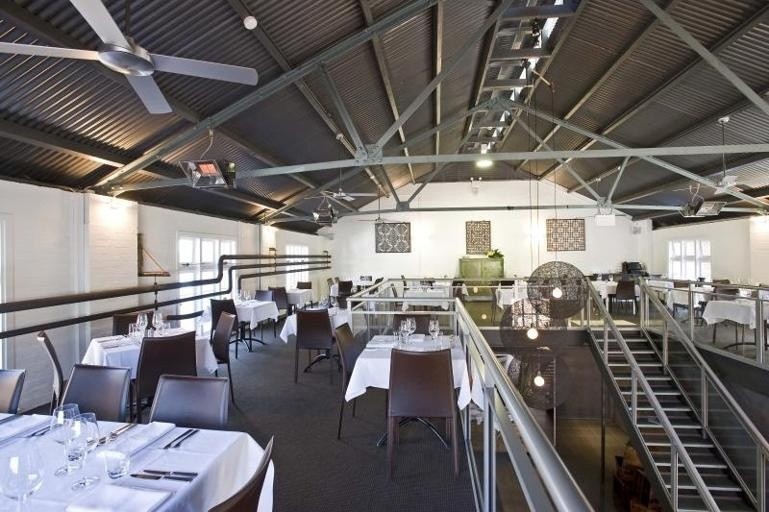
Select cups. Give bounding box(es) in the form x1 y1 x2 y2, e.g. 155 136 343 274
393 330 402 351
437 331 443 347
162 319 170 338
128 323 138 344
102 429 132 479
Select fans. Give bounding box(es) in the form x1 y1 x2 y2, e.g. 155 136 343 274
356 176 404 226
0 1 260 117
672 115 768 195
303 142 379 201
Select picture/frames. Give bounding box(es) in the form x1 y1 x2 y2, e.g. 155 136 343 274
546 218 586 251
465 221 491 255
374 222 411 252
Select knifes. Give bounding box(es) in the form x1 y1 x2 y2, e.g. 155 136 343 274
161 427 200 451
128 468 200 485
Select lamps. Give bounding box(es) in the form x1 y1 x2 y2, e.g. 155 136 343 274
230 1 259 32
526 83 588 318
311 197 339 225
177 128 237 191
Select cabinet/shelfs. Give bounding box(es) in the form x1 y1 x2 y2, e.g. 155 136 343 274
459 258 503 280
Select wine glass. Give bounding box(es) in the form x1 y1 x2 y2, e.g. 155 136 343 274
609 275 614 283
235 288 251 304
137 311 164 338
598 274 602 281
400 314 440 347
1 402 102 512
320 294 337 311
